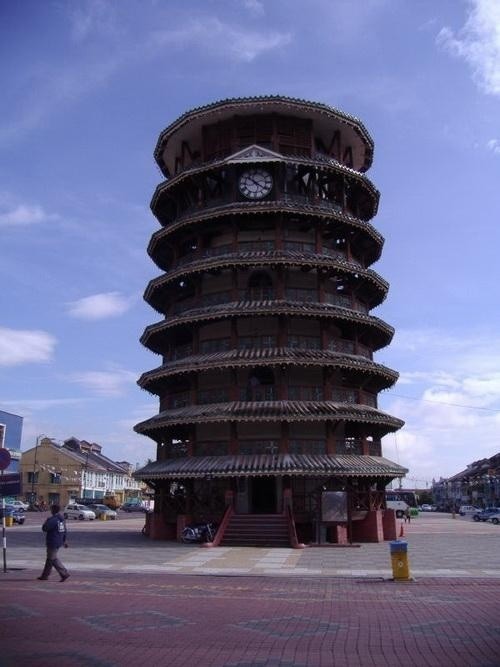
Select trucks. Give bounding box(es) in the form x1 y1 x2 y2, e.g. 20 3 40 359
75 495 121 510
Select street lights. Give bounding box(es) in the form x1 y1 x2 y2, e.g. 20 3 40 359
31 434 47 492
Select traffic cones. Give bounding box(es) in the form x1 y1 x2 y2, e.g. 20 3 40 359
398 522 406 537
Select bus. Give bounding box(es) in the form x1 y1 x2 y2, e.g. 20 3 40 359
385 489 420 517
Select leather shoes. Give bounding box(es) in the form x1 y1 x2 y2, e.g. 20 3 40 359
38 576 48 580
59 574 70 582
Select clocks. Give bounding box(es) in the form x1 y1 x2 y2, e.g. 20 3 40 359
238 168 273 200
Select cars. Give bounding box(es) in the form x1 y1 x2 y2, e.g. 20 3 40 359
123 503 149 513
63 504 117 520
0 501 29 524
420 504 500 524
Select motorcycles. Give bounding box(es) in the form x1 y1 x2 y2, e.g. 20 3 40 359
180 516 217 544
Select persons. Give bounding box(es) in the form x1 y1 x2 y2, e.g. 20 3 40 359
37 504 71 583
403 506 410 523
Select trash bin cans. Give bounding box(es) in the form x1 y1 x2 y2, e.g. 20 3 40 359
6 512 13 527
101 512 106 520
390 540 409 582
